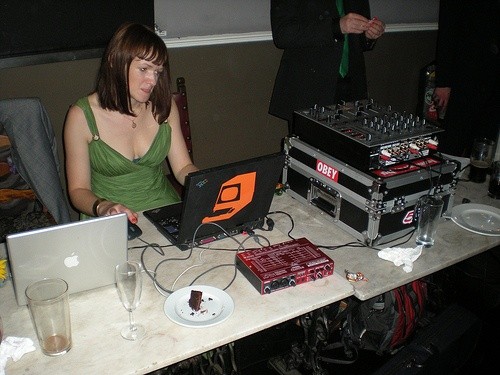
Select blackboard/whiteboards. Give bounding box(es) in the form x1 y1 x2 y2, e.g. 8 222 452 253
0 0 154 69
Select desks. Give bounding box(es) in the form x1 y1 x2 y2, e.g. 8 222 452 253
0 213 355 375
264 165 500 303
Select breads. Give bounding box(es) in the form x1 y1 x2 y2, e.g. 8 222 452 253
189 290 203 311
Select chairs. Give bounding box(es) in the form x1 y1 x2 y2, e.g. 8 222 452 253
0 96 73 242
165 77 195 167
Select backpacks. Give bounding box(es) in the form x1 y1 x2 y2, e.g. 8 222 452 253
346 275 426 356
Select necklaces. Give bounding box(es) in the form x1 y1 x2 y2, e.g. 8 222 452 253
116 102 148 128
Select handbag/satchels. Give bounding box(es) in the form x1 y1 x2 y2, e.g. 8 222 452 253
284 297 350 373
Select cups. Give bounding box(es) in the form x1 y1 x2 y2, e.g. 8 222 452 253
24 278 72 356
469 137 494 183
415 196 444 248
487 160 500 200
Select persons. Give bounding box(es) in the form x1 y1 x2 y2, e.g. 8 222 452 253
432 0 500 151
268 0 385 135
62 19 201 224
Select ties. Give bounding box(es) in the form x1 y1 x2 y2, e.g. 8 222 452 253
337 0 350 78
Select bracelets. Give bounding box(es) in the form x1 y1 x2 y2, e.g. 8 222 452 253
92 198 107 218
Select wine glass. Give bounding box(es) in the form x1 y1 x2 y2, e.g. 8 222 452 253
116 261 146 341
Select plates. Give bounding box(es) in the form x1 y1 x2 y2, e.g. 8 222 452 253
163 285 235 328
451 203 500 236
